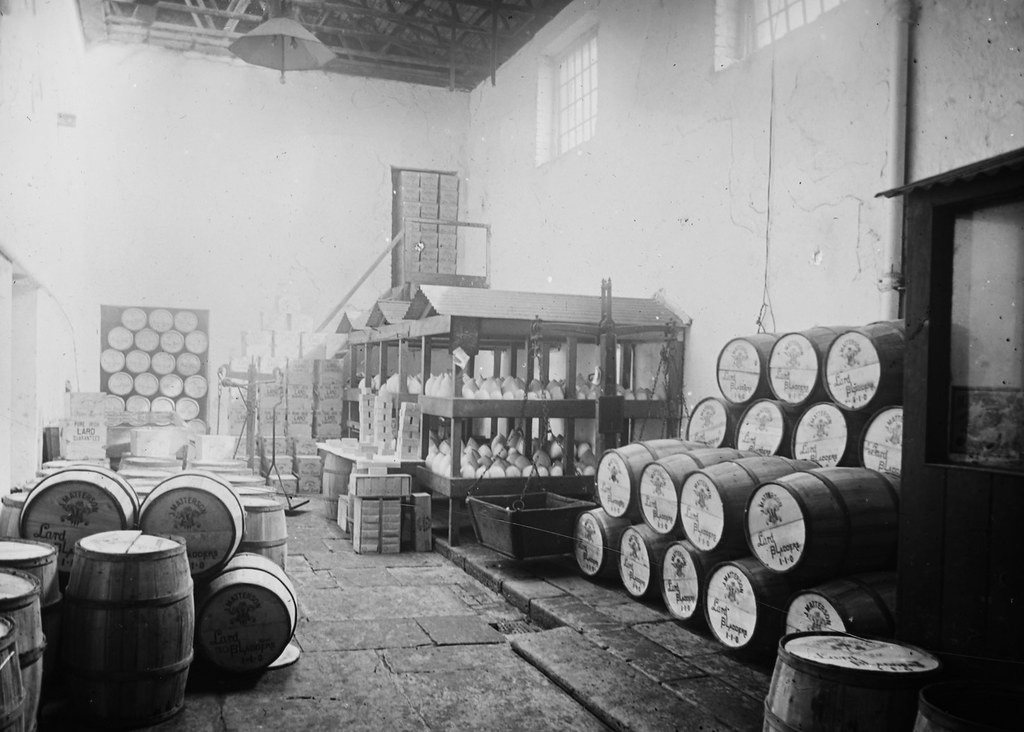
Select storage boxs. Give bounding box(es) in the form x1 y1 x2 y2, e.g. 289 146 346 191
247 359 432 554
58 392 107 460
195 435 237 461
129 427 179 460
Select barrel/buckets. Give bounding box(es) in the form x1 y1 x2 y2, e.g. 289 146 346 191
770 325 866 407
0 568 42 731
687 399 735 447
825 321 908 412
1 457 286 605
746 469 899 575
914 684 1024 732
639 449 765 535
663 542 724 623
716 332 778 404
762 632 940 732
574 509 630 580
792 404 861 469
861 407 903 487
787 587 938 639
1 614 23 732
598 439 706 518
197 552 296 672
620 525 679 599
682 456 819 551
738 398 792 457
705 558 804 650
67 530 195 716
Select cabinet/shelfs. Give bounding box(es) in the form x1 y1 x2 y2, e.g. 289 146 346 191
346 388 684 546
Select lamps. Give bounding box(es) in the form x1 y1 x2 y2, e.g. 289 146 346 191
226 0 338 85
